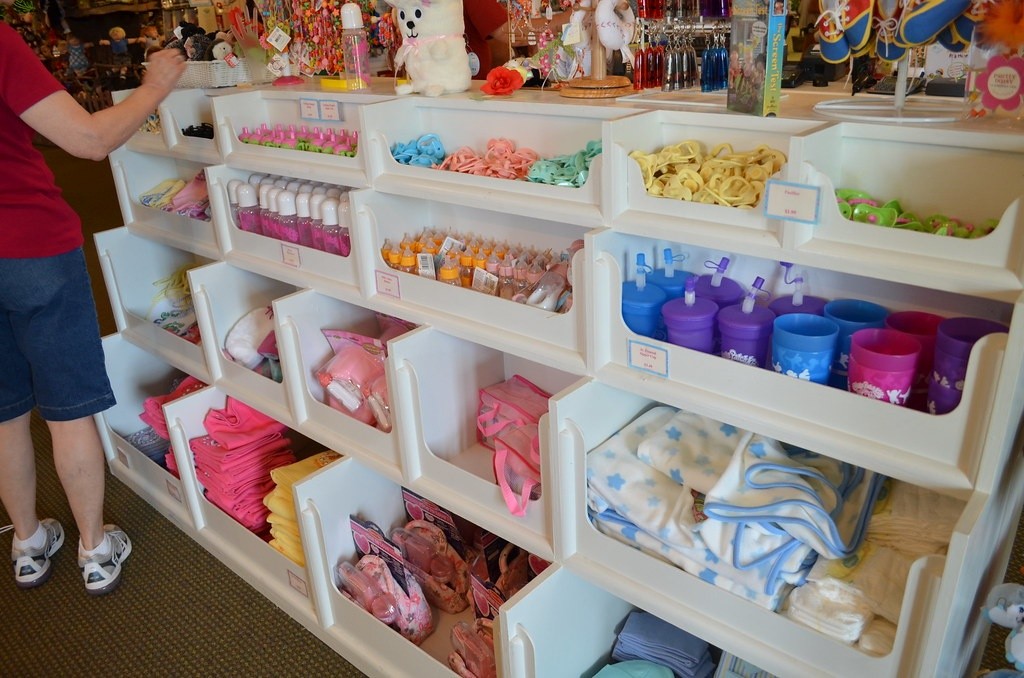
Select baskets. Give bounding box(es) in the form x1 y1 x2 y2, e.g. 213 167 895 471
142 54 291 88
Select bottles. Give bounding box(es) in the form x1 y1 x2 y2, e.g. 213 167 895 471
228 174 357 256
340 3 371 91
382 226 582 315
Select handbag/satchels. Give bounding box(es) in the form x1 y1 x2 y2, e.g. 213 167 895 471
476 374 555 517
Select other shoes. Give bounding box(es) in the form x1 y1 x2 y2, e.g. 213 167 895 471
813 0 991 88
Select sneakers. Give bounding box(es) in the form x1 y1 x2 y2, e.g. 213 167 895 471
0 518 64 587
77 523 133 595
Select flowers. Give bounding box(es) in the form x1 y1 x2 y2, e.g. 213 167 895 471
479 66 524 97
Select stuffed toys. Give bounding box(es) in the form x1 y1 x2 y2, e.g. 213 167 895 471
137 21 162 61
163 20 236 62
386 1 471 98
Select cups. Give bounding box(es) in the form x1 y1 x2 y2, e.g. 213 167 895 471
620 249 1007 415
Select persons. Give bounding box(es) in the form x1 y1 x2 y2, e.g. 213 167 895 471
97 26 140 81
62 33 94 74
0 20 188 595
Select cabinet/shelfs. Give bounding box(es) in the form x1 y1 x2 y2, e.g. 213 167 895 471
91 88 1024 677
